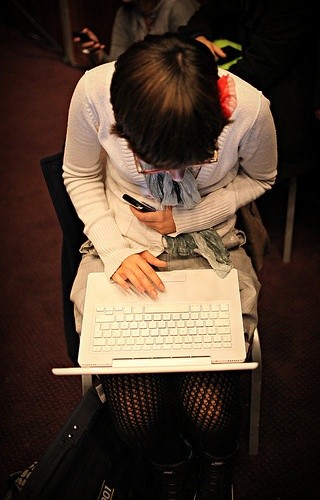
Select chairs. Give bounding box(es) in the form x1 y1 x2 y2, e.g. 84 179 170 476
40 149 262 455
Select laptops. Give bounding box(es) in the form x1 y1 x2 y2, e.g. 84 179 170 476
52 268 259 375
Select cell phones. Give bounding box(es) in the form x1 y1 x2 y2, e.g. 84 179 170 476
73 31 90 42
122 194 156 212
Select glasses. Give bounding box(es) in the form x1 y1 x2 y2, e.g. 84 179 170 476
132 141 219 175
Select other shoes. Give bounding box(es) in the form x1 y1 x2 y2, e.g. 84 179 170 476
195 439 237 500
151 448 192 500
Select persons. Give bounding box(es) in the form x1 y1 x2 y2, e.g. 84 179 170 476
178 0 320 161
72 0 202 73
62 37 277 500
